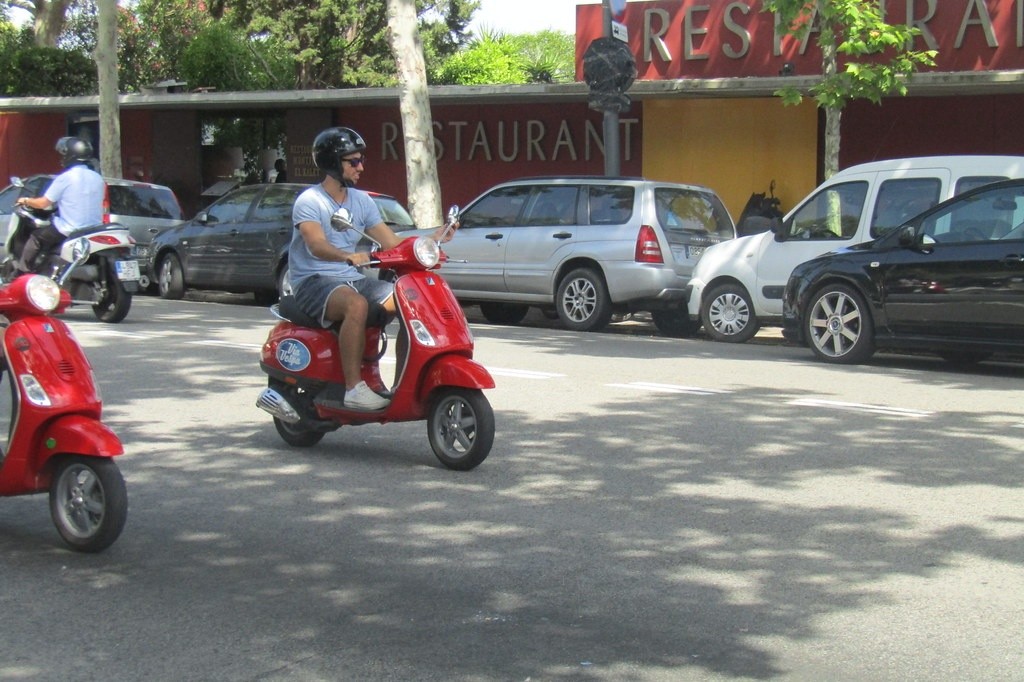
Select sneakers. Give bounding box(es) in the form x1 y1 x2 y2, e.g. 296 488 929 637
391 385 398 393
343 380 392 411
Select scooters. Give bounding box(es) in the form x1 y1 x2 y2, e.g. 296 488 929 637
737 180 786 239
3 175 150 323
256 204 496 471
1 238 128 553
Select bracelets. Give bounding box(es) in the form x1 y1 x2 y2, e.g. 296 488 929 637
24 197 29 205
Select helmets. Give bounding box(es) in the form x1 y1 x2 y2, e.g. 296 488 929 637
312 127 367 180
62 136 95 162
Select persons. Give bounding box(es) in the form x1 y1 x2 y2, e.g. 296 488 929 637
274 159 288 183
284 127 460 412
7 135 105 282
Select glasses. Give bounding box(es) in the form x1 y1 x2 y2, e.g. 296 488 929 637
340 154 365 168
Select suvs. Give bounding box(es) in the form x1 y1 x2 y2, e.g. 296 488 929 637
385 175 743 338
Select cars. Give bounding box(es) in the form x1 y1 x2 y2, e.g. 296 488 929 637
684 153 1024 368
146 182 418 308
0 173 188 296
